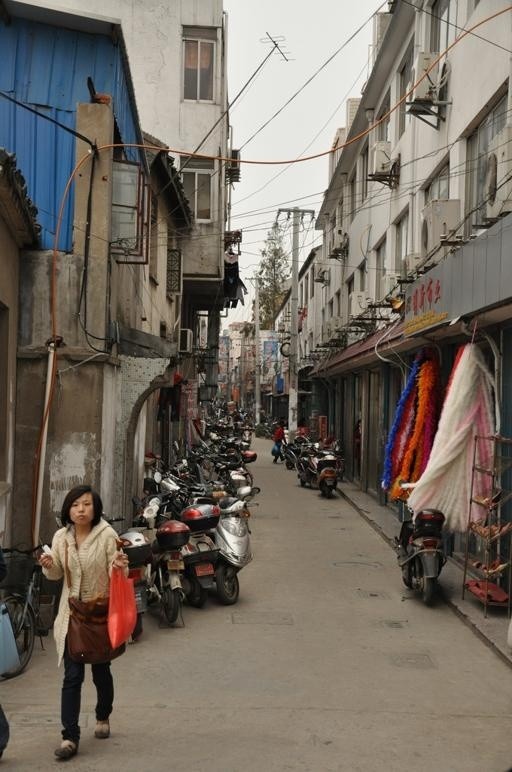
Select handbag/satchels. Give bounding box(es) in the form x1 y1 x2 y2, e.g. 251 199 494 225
67 594 128 663
0 605 23 678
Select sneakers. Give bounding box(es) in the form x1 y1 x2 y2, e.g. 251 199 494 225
50 738 79 760
94 719 111 741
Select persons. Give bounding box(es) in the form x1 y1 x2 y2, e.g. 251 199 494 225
0 544 11 761
232 411 240 435
273 422 288 464
38 484 130 758
240 411 247 422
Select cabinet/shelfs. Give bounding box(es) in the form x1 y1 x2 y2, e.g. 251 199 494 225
462 434 512 619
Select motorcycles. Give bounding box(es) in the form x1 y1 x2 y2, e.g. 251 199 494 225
282 434 345 498
261 413 285 439
393 497 448 606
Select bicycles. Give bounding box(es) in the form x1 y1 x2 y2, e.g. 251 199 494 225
1 544 52 679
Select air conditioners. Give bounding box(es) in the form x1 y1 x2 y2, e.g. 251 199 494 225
368 141 391 176
331 229 344 251
177 329 193 354
229 149 240 173
402 254 421 277
349 291 367 318
486 128 512 219
332 316 343 330
314 266 324 281
324 322 338 342
329 240 338 259
406 53 439 114
421 198 460 261
